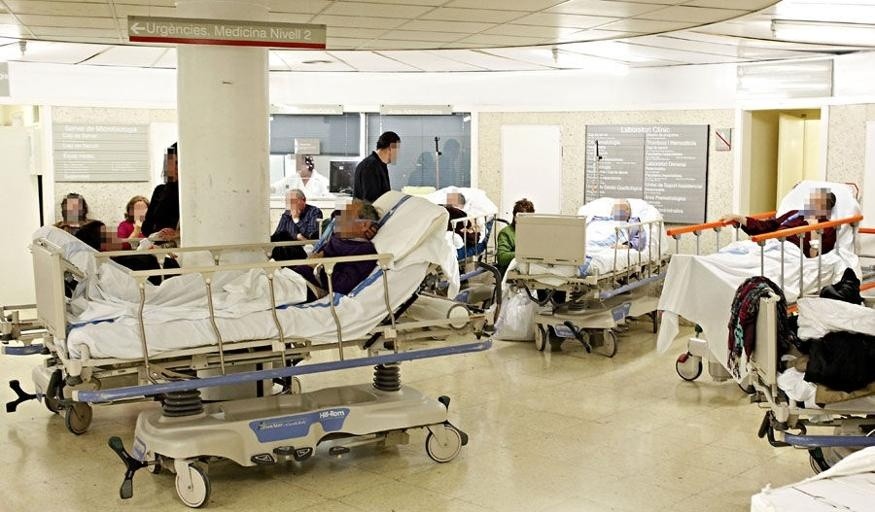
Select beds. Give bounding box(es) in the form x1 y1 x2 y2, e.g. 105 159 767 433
0 177 875 512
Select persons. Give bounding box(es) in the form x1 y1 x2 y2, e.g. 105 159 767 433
141 142 178 244
407 152 435 187
353 131 402 202
53 193 89 237
719 185 842 258
778 267 875 414
74 220 181 287
436 192 481 250
287 201 379 294
581 199 649 253
117 195 152 251
495 197 537 268
271 189 323 242
435 139 462 189
269 146 332 196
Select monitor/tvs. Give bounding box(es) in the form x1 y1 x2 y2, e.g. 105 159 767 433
329 161 358 196
